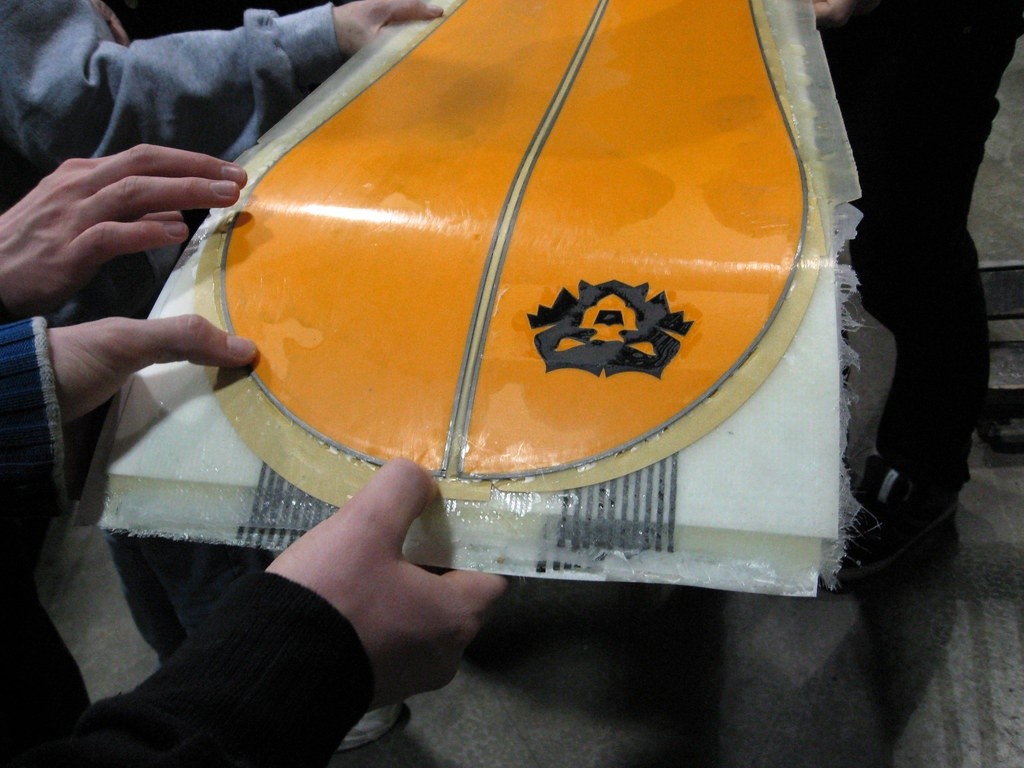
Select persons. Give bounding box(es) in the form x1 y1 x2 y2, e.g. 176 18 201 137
815 0 1024 593
1 2 506 768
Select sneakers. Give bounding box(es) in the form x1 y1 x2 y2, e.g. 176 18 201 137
835 455 959 579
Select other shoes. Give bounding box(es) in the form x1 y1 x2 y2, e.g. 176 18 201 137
336 702 410 753
